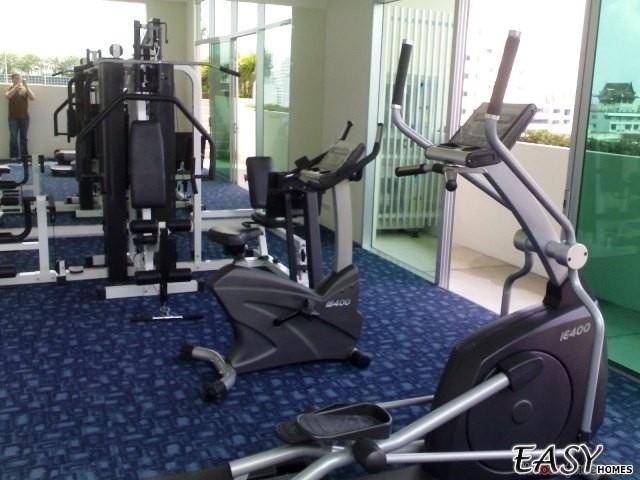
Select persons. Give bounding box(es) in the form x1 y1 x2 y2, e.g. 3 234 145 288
4 73 36 162
